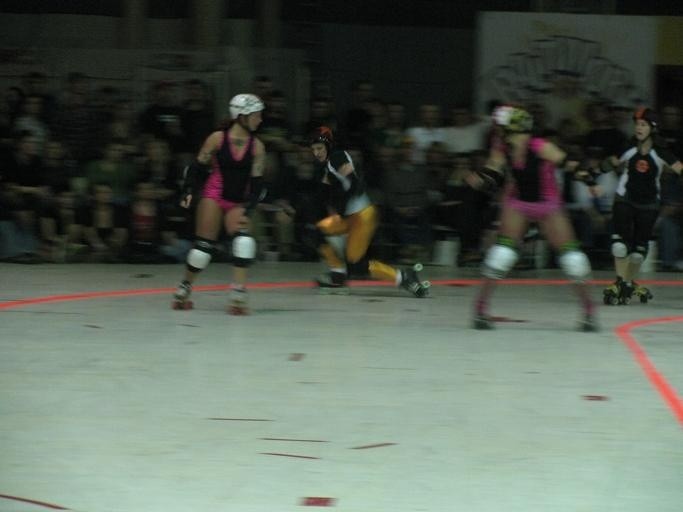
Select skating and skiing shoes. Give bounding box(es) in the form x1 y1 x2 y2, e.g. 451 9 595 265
168 279 195 312
399 262 431 299
629 281 653 304
225 286 249 315
312 272 352 296
470 312 599 331
601 283 634 306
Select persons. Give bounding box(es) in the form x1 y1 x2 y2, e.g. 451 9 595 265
305 124 428 299
424 105 607 329
171 92 267 311
583 106 682 300
0 71 683 275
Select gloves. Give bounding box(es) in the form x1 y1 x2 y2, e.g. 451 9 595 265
179 188 194 209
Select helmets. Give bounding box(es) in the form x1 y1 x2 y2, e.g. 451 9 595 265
305 131 333 146
226 93 265 120
632 106 660 128
493 106 534 132
